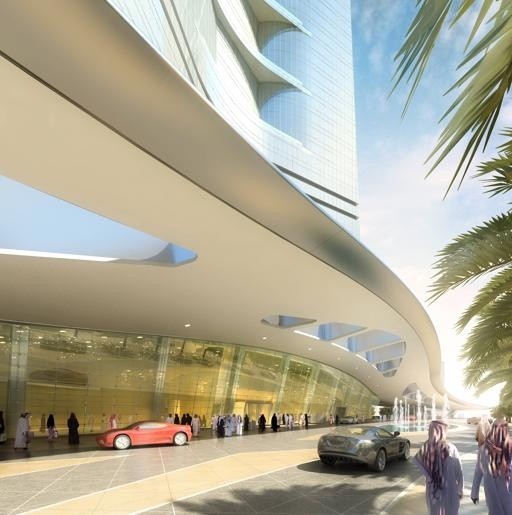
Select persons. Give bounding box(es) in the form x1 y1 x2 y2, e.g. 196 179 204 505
475 417 492 448
47 414 58 444
101 413 106 432
412 419 463 515
40 414 46 432
110 413 117 429
469 420 511 515
165 412 338 438
24 411 33 445
13 412 30 453
67 412 80 449
1 410 5 434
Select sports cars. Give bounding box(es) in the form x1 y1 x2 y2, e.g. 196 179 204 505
316 423 413 474
93 419 194 450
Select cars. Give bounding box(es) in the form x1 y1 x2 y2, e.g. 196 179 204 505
38 335 88 355
30 367 90 385
339 415 358 423
467 417 493 424
